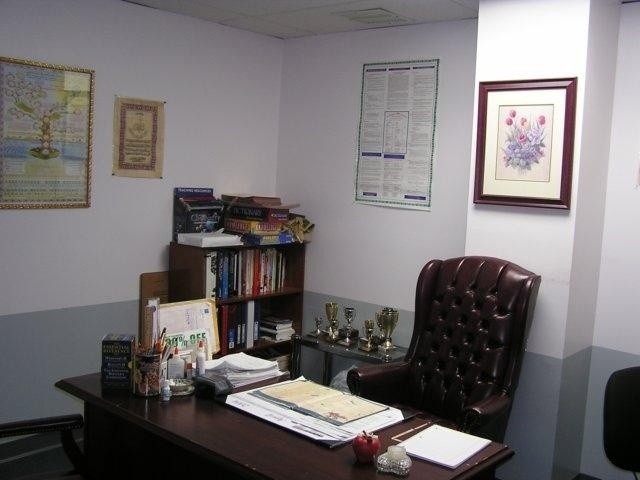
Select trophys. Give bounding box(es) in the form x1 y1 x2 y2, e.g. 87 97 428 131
309 301 399 354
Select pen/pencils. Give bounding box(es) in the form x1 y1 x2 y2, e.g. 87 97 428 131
152 328 169 354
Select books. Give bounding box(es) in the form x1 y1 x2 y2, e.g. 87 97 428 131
397 422 492 470
251 378 388 428
173 186 296 350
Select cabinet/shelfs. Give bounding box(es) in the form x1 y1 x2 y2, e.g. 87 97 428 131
169 241 310 370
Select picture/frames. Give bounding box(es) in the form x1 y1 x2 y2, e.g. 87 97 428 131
473 77 578 209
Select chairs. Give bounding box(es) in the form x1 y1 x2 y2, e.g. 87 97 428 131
604 366 640 480
347 256 542 444
0 414 85 480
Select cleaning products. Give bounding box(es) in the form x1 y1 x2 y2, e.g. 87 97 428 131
167 339 206 378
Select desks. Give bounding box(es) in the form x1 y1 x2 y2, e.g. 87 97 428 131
290 326 408 386
54 371 516 480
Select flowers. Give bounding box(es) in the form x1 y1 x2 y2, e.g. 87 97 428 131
502 109 546 170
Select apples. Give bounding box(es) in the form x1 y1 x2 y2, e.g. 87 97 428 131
352 430 380 464
